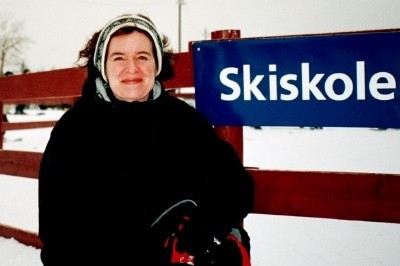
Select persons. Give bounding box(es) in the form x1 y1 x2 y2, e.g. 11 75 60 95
37 13 255 266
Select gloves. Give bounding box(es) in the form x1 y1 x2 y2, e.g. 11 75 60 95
177 202 236 256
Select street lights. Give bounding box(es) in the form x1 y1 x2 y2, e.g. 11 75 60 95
175 0 187 53
